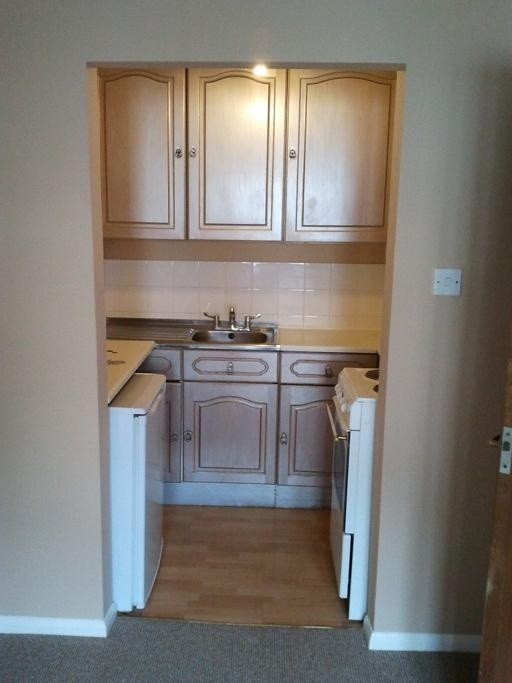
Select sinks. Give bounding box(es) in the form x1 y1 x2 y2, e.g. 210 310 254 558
185 328 278 346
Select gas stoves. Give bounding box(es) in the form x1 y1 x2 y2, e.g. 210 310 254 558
343 366 379 401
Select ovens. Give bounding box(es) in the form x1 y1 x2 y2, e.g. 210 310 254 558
324 395 350 533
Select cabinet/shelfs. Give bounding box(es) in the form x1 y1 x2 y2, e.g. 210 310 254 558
275 350 378 509
95 68 286 263
283 69 398 266
137 344 281 510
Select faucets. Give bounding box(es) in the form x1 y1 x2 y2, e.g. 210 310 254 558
228 306 235 324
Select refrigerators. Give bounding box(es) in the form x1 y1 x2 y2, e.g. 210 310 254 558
109 371 168 613
